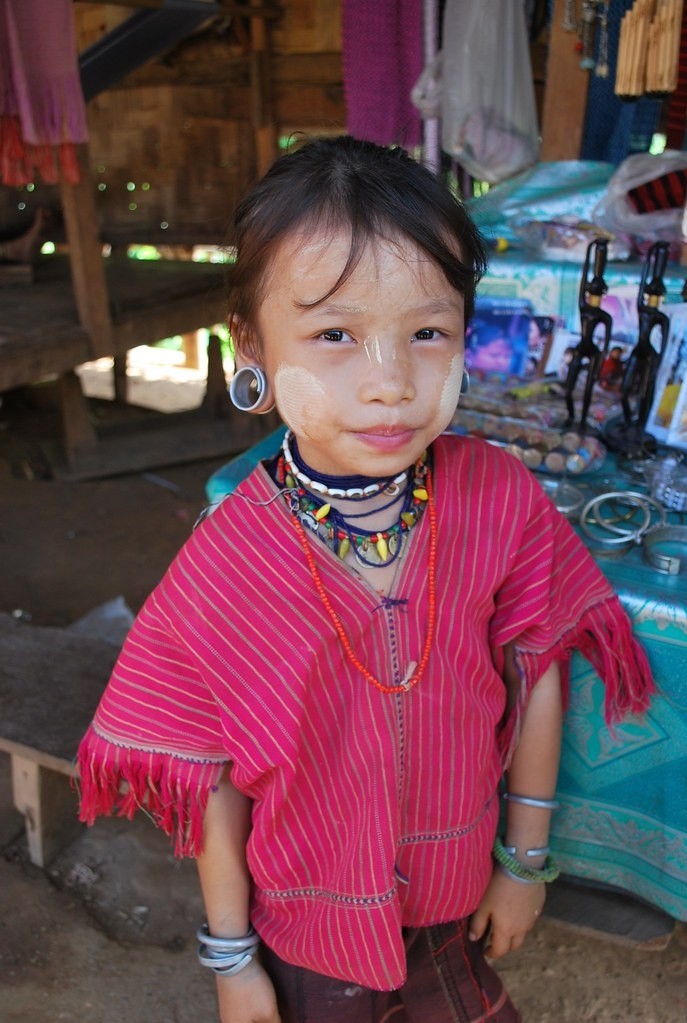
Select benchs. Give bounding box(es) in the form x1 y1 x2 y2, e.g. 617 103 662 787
0 625 124 867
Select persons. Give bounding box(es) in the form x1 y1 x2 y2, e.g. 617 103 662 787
562 237 612 433
599 346 625 391
619 239 671 436
556 345 575 382
72 135 656 1023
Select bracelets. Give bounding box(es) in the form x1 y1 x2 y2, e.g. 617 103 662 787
195 921 261 977
492 837 560 885
502 793 560 808
566 466 669 546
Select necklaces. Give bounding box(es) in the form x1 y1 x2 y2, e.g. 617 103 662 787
267 427 437 697
